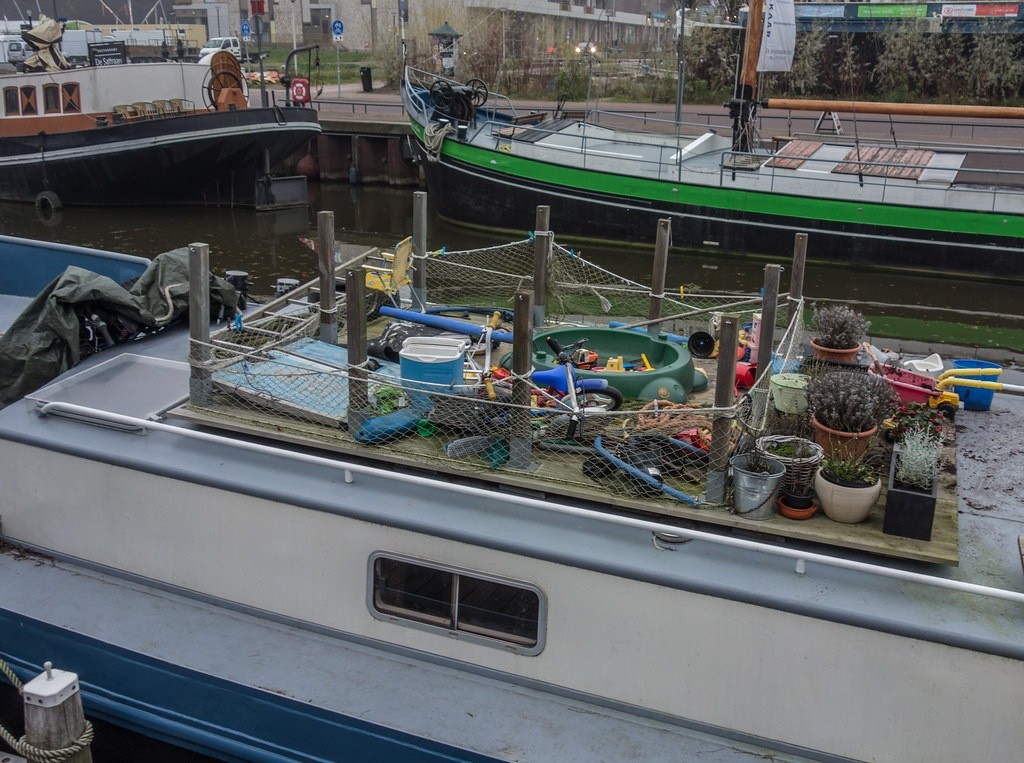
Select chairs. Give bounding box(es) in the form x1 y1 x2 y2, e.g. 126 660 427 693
113 99 196 123
361 235 427 316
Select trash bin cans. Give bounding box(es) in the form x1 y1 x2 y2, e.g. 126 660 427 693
359 66 372 93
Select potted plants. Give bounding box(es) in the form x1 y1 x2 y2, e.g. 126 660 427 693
729 441 786 521
810 303 872 363
803 367 901 460
880 424 948 542
782 418 816 509
814 419 884 524
755 435 824 489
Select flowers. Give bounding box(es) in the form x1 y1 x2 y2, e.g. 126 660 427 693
888 400 945 442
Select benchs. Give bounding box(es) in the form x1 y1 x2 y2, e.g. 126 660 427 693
491 112 548 149
556 111 590 123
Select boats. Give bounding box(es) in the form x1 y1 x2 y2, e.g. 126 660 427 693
1 0 322 207
403 0 1024 285
0 230 1023 762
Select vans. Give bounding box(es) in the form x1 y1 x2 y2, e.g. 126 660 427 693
7 40 36 61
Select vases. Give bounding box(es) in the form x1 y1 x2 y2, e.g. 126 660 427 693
776 496 817 520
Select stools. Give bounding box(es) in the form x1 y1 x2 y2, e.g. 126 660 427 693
771 136 799 154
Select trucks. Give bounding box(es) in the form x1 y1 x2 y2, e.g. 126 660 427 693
199 36 269 63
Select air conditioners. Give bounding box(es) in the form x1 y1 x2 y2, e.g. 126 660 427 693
915 17 942 33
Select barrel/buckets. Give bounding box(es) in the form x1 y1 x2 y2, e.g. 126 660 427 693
770 373 821 413
730 453 786 520
952 359 1003 410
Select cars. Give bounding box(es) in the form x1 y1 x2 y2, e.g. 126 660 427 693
576 41 596 54
